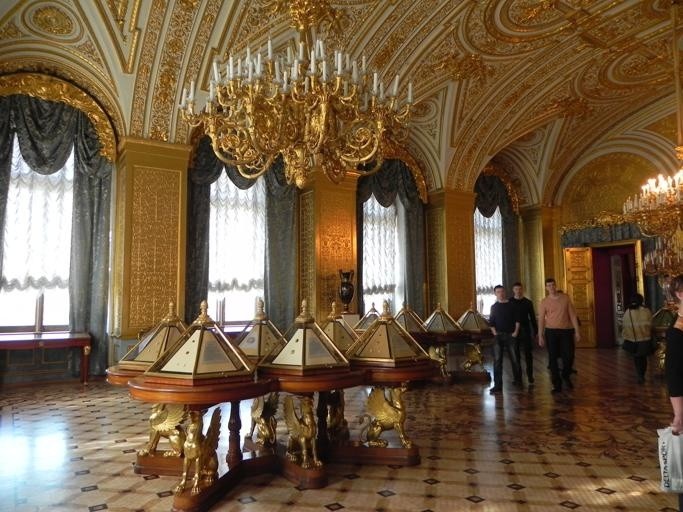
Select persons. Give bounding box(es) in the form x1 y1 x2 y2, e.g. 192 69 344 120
537 278 582 394
508 281 538 386
488 285 523 393
622 292 657 383
664 274 683 436
547 289 582 375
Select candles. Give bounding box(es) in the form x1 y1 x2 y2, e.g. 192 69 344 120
215 296 225 326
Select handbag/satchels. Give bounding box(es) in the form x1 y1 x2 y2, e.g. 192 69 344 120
623 339 637 352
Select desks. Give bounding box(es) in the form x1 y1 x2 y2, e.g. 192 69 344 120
0 331 92 387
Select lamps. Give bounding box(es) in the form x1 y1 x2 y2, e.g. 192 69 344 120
177 1 417 194
622 1 683 276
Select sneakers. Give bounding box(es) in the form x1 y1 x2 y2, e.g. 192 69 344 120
490 376 534 393
550 374 576 394
